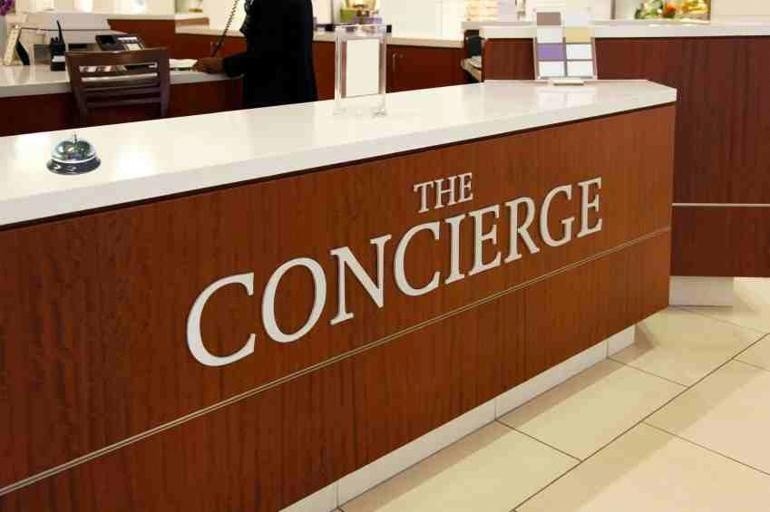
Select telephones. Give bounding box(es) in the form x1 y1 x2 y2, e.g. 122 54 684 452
95 35 155 71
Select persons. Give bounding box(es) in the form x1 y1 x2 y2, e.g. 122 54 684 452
194 1 317 111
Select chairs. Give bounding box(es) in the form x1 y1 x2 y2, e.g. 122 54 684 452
64 46 171 125
